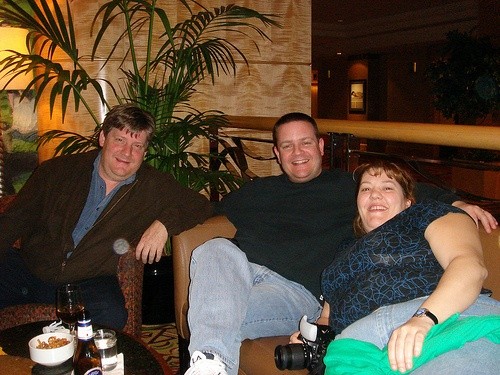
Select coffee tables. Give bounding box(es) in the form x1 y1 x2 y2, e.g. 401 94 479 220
0 321 173 375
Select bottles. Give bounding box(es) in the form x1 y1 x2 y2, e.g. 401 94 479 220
73 309 104 375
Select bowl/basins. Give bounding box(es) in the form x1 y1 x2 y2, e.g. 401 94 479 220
29 333 75 367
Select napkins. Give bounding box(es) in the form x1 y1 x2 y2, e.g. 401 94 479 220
72 350 124 375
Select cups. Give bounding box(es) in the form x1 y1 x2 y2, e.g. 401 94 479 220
93 328 118 371
360 143 368 151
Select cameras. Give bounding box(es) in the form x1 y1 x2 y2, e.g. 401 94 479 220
272 322 335 375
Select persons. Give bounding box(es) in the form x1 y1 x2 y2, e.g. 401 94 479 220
0 103 214 331
288 159 500 375
184 112 498 375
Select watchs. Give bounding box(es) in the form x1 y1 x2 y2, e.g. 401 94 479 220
412 308 438 325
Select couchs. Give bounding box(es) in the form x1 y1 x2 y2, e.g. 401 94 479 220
174 215 500 375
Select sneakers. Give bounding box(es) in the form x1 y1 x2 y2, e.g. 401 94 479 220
184 350 227 375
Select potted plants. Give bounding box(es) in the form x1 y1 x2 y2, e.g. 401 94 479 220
0 0 277 321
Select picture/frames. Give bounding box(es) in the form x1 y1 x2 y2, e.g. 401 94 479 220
349 80 366 113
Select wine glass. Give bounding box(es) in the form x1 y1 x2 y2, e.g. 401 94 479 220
57 284 85 333
